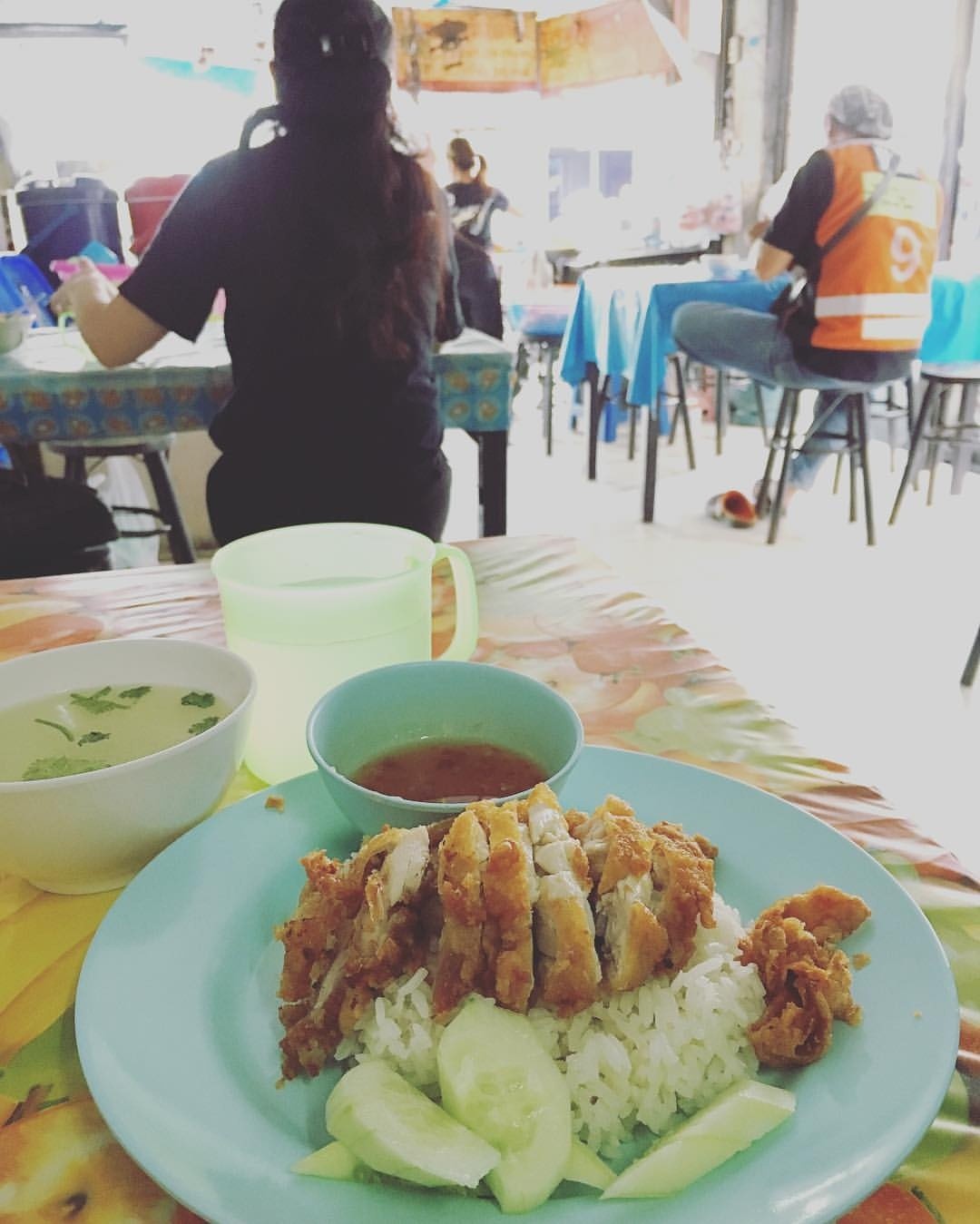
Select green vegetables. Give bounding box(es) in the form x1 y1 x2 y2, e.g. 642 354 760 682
7 676 222 792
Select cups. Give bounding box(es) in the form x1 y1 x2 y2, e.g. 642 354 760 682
211 521 480 793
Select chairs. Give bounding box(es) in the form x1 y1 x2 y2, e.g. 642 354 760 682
512 218 979 545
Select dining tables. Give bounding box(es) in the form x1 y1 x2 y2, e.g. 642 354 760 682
2 522 978 1221
0 292 514 536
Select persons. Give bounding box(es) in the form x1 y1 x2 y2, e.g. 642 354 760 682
437 136 521 343
669 80 945 516
55 0 464 553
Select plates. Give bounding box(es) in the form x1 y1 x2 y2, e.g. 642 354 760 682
76 743 961 1224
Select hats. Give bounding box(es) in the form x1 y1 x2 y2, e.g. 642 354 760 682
276 1 391 66
830 85 894 140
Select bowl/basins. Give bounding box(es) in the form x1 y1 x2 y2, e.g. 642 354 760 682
701 252 741 280
1 632 264 899
1 311 34 356
305 657 588 844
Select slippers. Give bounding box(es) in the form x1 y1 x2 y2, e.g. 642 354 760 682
706 489 756 528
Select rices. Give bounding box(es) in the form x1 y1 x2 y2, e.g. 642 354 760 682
309 884 774 1154
